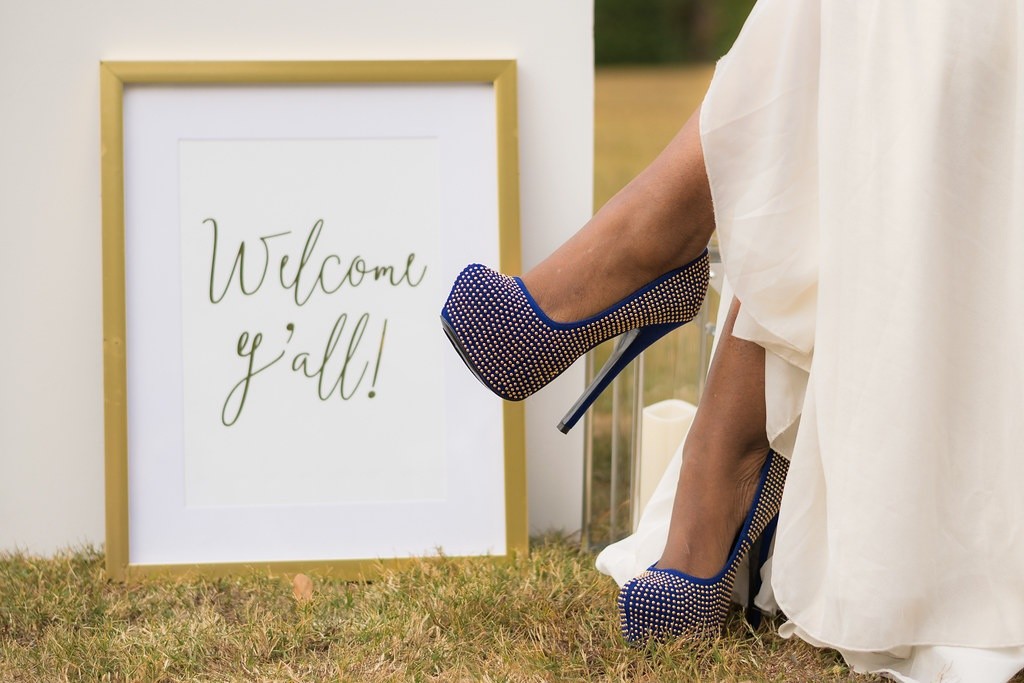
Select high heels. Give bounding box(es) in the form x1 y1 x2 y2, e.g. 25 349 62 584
619 449 791 646
437 246 711 434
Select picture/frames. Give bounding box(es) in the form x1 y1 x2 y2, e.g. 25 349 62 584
97 56 532 585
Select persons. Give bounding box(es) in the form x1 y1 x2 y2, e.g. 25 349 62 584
440 0 1024 683
